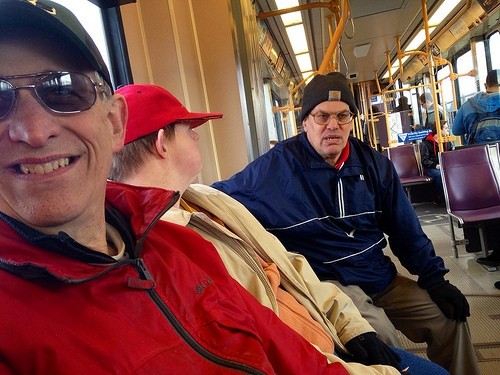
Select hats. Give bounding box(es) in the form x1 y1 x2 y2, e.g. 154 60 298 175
114 84 224 144
301 72 358 121
0 0 114 93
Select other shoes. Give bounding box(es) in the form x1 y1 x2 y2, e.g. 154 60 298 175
476 252 500 267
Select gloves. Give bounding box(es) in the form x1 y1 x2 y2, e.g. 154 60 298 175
345 332 402 372
425 274 470 322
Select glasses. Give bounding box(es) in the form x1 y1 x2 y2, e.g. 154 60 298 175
310 111 355 125
420 102 425 106
0 71 103 120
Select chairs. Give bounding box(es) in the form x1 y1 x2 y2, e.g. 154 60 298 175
377 112 435 209
438 140 500 271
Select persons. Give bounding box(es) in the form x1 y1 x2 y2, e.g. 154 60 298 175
420 120 456 207
391 96 417 144
451 68 500 174
363 104 382 146
209 71 480 375
474 221 500 293
411 93 446 143
0 0 352 375
109 84 448 375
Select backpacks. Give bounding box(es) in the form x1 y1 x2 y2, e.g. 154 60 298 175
464 96 500 145
